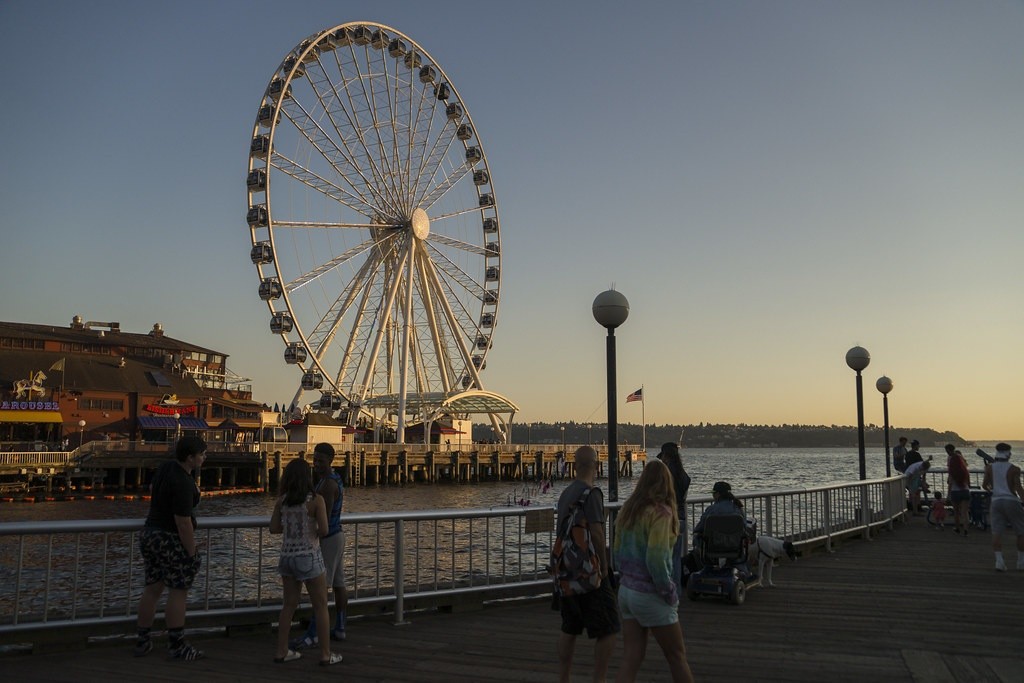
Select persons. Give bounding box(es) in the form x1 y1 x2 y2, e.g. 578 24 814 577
944 444 971 537
602 438 605 445
268 458 343 667
132 435 207 663
612 458 696 683
982 442 1024 572
555 446 622 683
289 443 346 653
893 438 908 473
58 437 69 452
419 437 427 455
903 460 930 513
103 431 111 441
472 437 503 453
693 482 753 578
8 442 15 452
445 439 452 452
655 442 691 597
905 439 923 467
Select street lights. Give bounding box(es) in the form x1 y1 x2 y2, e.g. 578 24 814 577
78 419 85 448
526 422 532 454
173 412 180 442
560 426 565 445
845 345 870 508
586 425 592 445
458 421 462 451
491 427 494 443
592 289 631 546
876 376 893 478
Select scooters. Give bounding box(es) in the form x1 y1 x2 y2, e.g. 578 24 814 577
686 517 761 606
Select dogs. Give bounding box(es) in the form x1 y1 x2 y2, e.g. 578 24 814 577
747 535 799 588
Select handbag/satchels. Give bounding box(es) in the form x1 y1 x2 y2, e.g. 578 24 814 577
546 485 606 597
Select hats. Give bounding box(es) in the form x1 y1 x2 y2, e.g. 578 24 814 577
656 442 677 458
995 447 1010 458
708 481 731 494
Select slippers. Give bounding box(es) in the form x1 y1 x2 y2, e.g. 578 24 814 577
273 650 302 662
319 652 343 666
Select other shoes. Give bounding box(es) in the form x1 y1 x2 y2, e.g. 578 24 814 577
1016 560 1024 569
996 561 1007 570
952 528 961 535
960 529 969 536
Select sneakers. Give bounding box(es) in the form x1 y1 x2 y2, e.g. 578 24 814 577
288 633 320 650
135 639 153 657
166 642 204 661
330 627 345 640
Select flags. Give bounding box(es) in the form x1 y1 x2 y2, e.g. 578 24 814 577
626 388 642 402
